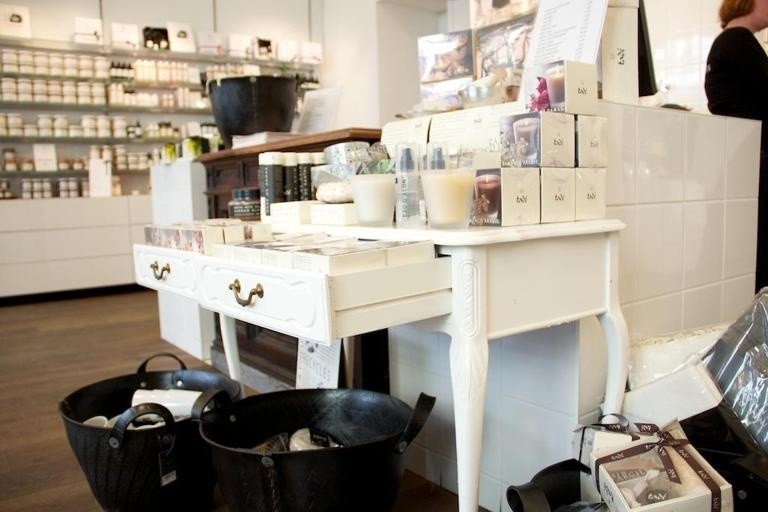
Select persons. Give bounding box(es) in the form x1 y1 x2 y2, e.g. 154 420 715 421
704 0 768 292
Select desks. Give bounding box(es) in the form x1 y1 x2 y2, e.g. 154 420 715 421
129 212 631 512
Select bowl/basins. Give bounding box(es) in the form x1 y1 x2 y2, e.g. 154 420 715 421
457 83 522 108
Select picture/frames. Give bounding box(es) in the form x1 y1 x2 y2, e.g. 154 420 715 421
472 9 538 80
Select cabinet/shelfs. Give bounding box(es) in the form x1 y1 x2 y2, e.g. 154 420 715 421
0 34 317 179
0 194 157 306
192 127 387 398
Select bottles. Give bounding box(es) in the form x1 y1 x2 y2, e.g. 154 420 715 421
228 188 263 223
110 58 203 171
395 144 427 229
112 176 124 199
204 62 255 95
259 150 325 216
0 49 113 202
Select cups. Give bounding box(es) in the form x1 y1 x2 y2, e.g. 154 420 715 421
544 66 567 113
348 174 397 227
475 174 500 222
512 119 539 165
